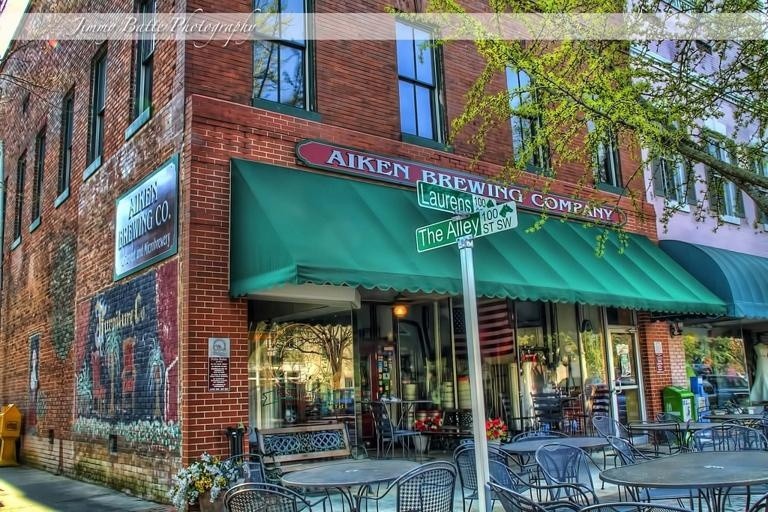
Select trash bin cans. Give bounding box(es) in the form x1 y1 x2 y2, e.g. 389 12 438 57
663 385 697 447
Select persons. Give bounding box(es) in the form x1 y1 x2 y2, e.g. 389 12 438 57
748 342 768 403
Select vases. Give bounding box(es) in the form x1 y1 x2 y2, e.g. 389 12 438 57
199 488 229 512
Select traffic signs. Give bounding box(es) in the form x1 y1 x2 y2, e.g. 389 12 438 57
414 202 519 252
417 181 499 219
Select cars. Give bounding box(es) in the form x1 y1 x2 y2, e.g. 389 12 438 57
263 383 403 423
547 371 751 419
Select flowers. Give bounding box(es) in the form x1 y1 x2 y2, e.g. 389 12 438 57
168 452 244 510
